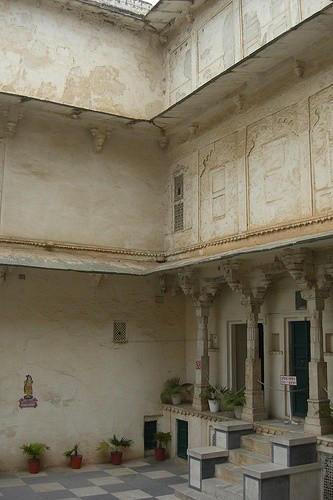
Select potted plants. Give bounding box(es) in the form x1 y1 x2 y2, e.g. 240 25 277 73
60 439 84 471
95 433 135 467
152 429 171 462
14 439 51 475
197 384 230 413
159 375 193 405
222 388 246 419
